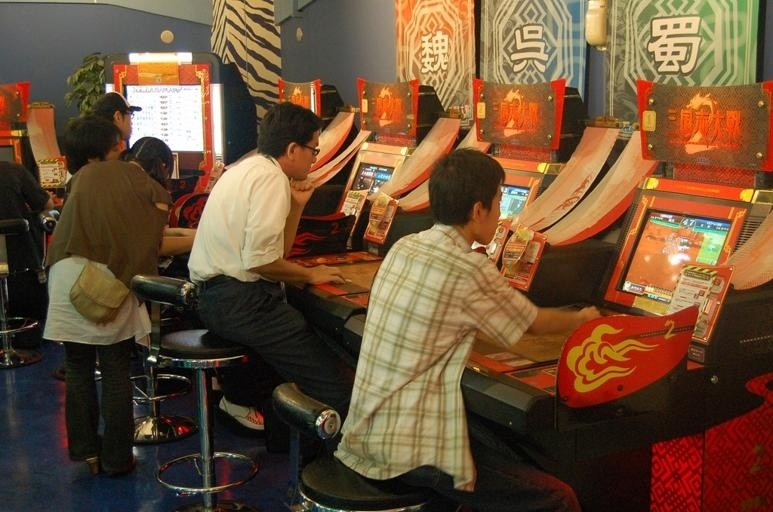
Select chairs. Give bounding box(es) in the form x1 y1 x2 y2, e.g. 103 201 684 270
0 219 43 369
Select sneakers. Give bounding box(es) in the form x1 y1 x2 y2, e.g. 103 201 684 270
211 376 265 430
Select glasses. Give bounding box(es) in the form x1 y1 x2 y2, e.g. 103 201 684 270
122 112 135 118
298 143 320 156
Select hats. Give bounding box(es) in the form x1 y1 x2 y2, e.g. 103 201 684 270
101 93 142 111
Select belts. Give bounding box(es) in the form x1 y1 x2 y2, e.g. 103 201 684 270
203 274 235 288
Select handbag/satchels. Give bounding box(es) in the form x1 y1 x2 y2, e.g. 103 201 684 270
69 264 130 323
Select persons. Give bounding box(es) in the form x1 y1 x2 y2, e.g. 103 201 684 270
0 161 54 350
64 118 264 430
332 148 602 512
187 102 353 456
91 93 142 140
42 137 174 477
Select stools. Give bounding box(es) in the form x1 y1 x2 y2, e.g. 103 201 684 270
129 272 259 511
272 382 427 512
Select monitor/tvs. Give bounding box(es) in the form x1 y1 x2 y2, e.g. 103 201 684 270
621 211 731 303
350 163 395 195
0 146 14 163
499 184 530 222
